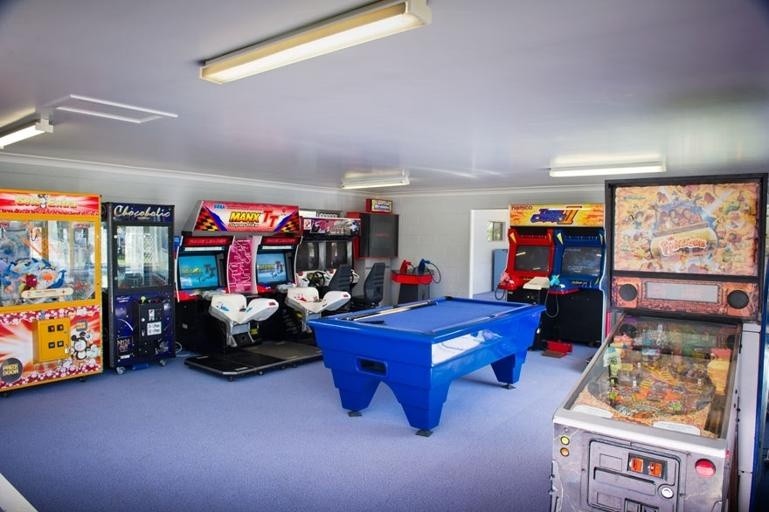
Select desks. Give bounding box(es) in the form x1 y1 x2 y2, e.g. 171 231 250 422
306 295 547 437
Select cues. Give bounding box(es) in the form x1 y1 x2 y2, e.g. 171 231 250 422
344 300 438 321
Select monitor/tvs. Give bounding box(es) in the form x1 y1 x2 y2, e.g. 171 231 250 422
326 241 347 269
361 213 398 257
562 246 600 276
255 252 288 281
177 254 221 290
514 244 549 271
296 241 320 271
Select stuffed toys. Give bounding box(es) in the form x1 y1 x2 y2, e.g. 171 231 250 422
0 256 67 305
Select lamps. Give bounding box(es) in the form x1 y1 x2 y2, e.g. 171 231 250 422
199 0 433 86
338 172 411 191
0 119 54 150
548 160 667 178
